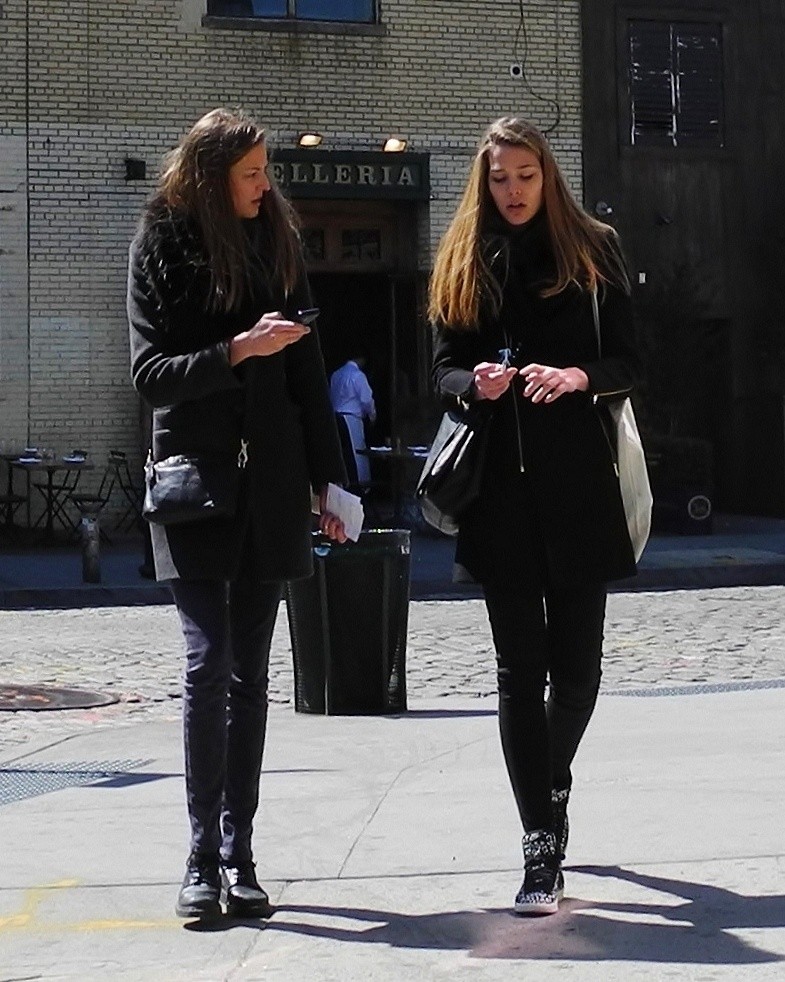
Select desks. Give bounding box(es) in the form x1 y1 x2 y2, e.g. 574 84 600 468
355 445 429 507
0 455 95 545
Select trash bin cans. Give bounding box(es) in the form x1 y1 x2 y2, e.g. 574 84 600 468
286 529 413 715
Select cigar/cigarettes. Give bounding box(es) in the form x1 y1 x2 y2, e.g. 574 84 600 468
501 361 506 372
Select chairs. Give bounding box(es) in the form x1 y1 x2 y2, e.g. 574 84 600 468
0 494 28 540
30 450 88 533
112 450 145 532
65 453 126 548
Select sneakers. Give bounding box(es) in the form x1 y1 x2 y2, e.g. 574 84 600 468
551 789 571 855
514 827 568 914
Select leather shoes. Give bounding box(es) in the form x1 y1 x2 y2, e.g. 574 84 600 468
175 850 223 917
218 855 268 911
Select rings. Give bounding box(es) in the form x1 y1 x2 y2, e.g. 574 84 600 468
322 525 328 530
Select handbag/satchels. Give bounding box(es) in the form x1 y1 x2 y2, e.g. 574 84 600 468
585 250 655 582
142 447 240 528
414 410 490 537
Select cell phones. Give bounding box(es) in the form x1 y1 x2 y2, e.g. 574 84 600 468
293 307 320 326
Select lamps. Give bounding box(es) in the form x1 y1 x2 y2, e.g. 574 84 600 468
384 139 407 153
297 132 324 147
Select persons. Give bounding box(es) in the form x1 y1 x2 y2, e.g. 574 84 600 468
331 347 376 499
427 116 634 913
125 105 351 923
